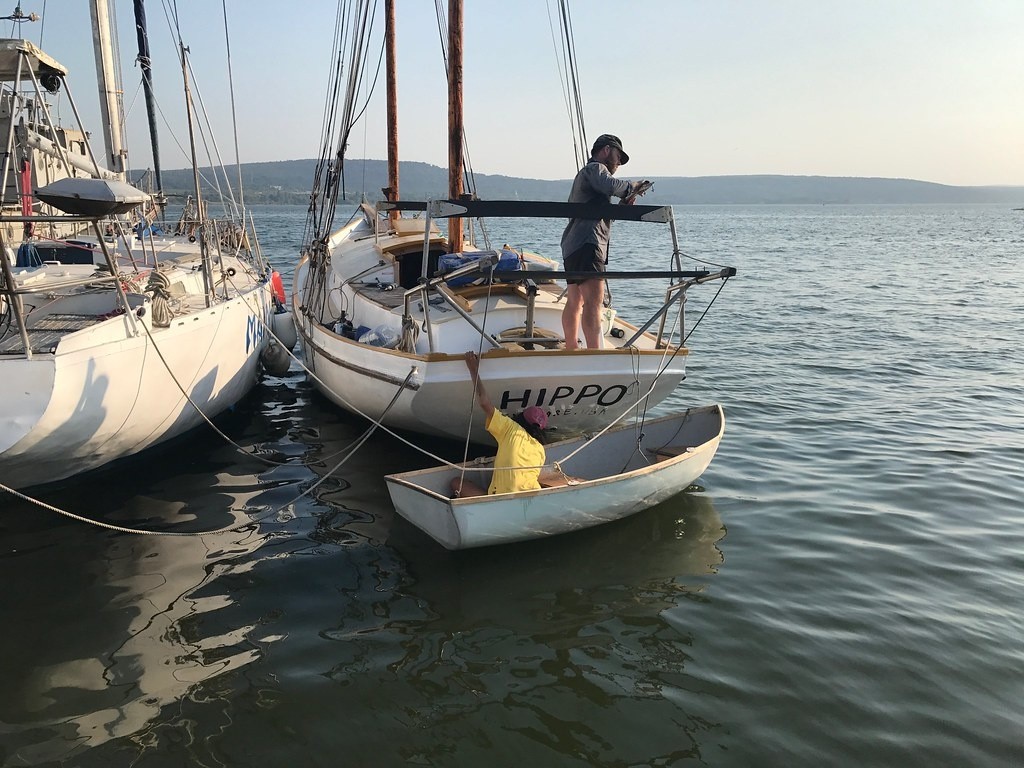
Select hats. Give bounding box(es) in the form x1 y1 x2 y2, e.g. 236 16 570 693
522 406 548 430
591 133 630 165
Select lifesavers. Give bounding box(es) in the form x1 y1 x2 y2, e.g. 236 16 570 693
271 271 286 304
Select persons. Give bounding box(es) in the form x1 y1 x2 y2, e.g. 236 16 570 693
450 351 549 500
562 135 650 349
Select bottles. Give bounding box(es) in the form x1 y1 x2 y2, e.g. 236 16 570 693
358 325 399 349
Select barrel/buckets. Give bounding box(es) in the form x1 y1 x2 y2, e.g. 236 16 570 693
600 308 617 337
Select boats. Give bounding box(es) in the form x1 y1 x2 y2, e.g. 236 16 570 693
382 404 724 550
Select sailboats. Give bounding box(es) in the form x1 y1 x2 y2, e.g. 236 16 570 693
293 0 691 446
0 0 275 494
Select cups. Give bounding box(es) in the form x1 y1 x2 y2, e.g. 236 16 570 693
610 328 624 338
